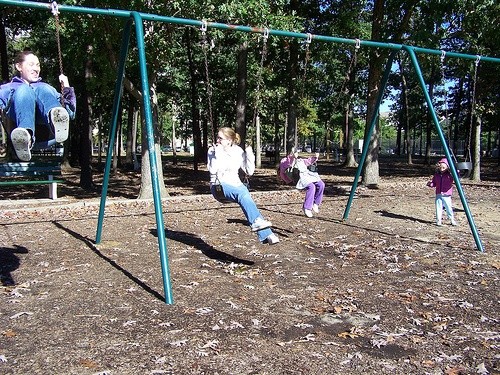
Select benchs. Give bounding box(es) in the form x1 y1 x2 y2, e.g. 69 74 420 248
0 158 63 200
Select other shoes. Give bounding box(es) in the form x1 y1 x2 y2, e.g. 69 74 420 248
437 221 440 226
451 221 456 225
11 128 31 161
313 203 320 213
51 107 69 142
302 208 313 218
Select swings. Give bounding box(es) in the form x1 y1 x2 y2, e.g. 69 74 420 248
440 59 480 178
202 34 266 204
0 13 72 164
285 44 358 187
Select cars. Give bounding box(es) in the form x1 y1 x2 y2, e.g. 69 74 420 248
93 146 99 152
163 146 172 152
137 144 142 152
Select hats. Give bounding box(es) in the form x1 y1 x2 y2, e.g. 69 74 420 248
439 158 450 168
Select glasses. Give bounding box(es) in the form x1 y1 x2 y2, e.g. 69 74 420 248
216 137 228 140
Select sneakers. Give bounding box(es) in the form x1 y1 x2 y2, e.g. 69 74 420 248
251 218 272 231
267 234 279 245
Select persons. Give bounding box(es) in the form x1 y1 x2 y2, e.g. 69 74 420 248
427 158 457 226
0 51 76 162
207 127 280 245
277 147 325 218
